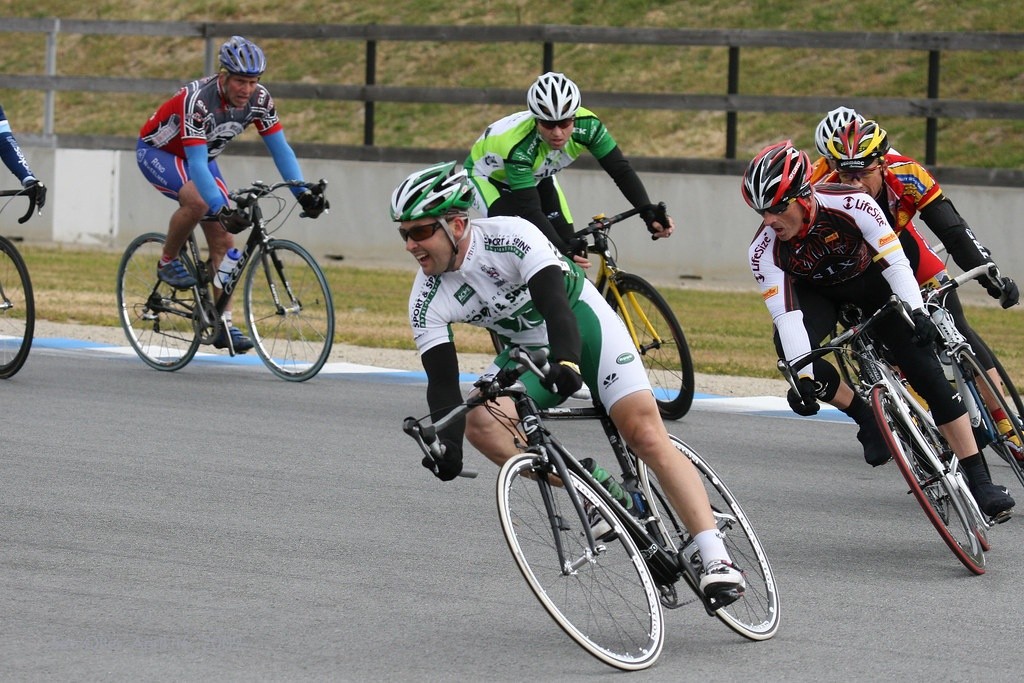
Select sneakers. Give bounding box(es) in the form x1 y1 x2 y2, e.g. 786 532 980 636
157 259 197 289
584 500 610 539
213 328 254 350
700 561 745 599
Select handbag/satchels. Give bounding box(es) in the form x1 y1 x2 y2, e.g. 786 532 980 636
27 178 46 210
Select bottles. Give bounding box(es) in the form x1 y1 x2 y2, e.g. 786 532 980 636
213 248 243 289
620 473 646 514
579 457 634 511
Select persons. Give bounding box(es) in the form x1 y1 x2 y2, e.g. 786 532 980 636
462 71 673 273
390 160 746 601
136 35 317 352
770 105 1024 467
740 138 1016 522
0 101 47 209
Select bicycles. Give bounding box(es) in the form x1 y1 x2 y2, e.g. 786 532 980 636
915 241 1024 490
116 178 336 383
775 292 1015 575
401 345 783 671
0 180 49 381
489 201 696 423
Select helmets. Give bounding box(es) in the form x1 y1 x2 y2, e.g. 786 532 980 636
218 36 266 76
526 71 582 122
815 106 869 158
389 160 477 222
741 140 813 210
826 120 889 170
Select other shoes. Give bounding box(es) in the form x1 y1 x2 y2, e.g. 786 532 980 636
856 428 890 465
974 483 1014 515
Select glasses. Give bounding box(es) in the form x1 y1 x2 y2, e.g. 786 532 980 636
538 118 573 129
836 163 882 181
756 186 810 217
397 216 460 242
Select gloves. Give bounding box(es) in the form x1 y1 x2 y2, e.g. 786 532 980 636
986 277 1019 309
539 361 582 398
216 207 252 234
297 191 330 219
787 377 821 417
905 313 938 345
421 444 462 481
